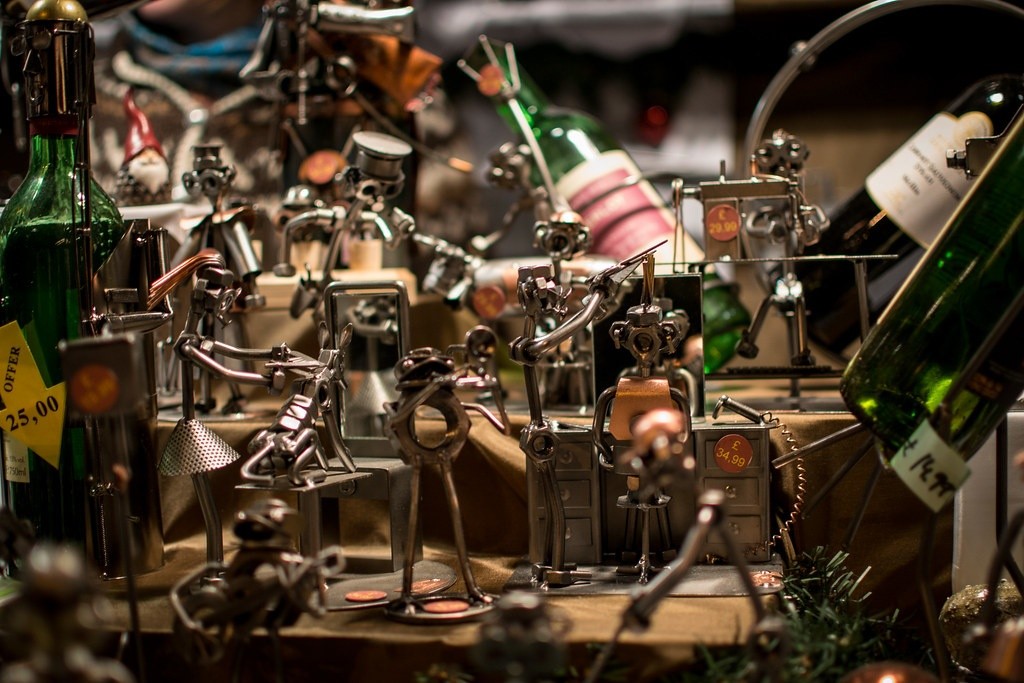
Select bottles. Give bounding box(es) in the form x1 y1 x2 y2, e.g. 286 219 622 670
474 52 751 375
839 109 1024 466
0 0 168 575
774 73 1023 352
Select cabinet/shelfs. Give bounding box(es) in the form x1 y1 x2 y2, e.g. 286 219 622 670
529 416 775 566
